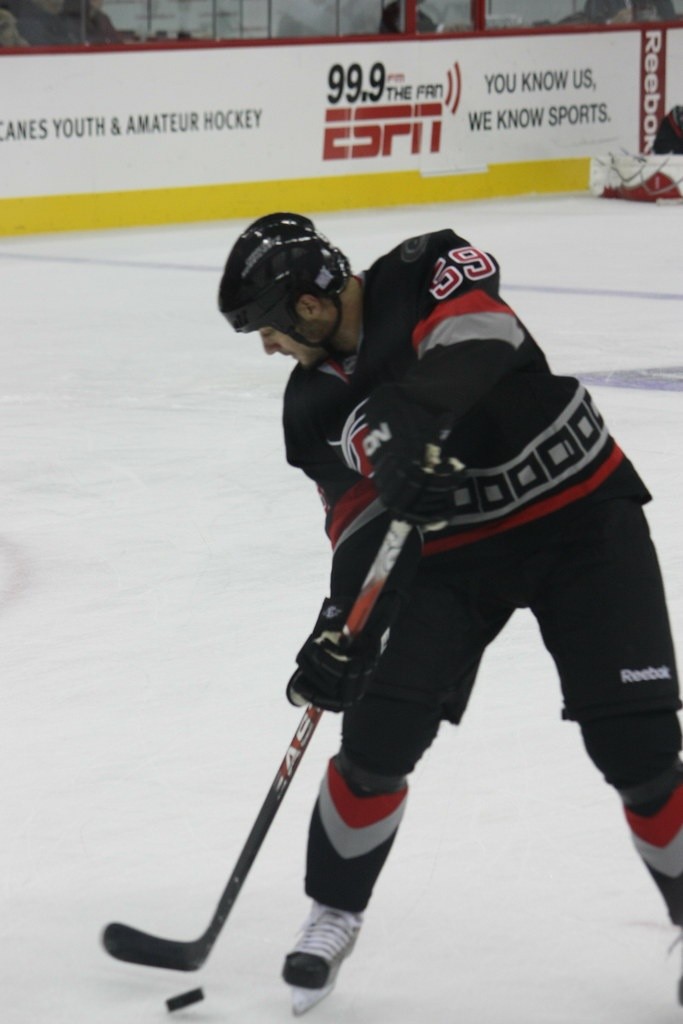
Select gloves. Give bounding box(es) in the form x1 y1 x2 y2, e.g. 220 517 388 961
286 597 396 712
364 385 458 526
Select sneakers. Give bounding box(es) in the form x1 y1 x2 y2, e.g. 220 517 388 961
282 898 363 1017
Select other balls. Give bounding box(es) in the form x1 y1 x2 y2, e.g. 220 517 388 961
167 985 204 1013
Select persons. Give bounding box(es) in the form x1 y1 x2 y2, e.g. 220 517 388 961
218 213 683 1017
0 0 136 47
380 0 435 33
585 0 676 24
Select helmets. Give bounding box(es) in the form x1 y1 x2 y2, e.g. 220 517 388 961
217 213 351 333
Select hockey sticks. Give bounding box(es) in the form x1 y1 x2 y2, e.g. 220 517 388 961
101 426 447 972
